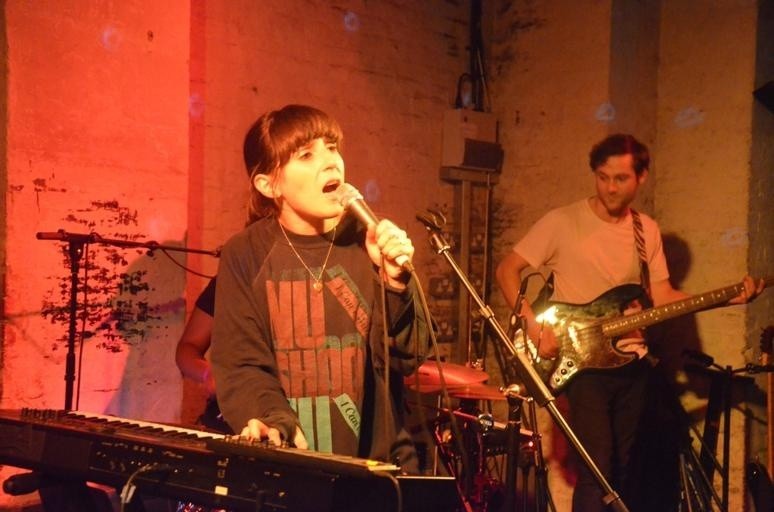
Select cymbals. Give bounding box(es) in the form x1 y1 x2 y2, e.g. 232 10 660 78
444 384 517 400
403 360 487 393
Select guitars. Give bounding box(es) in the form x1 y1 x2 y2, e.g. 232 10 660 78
505 277 769 402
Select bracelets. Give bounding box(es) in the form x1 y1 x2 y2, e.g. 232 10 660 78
203 363 212 382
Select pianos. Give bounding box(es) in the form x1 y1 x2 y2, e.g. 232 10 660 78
0 407 408 508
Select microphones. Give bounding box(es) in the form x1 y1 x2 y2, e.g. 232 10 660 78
336 183 415 273
507 277 527 342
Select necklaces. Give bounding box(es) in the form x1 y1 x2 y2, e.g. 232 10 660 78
276 214 337 294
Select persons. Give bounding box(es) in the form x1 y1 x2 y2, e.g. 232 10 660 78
175 273 236 437
493 133 765 512
209 104 441 478
